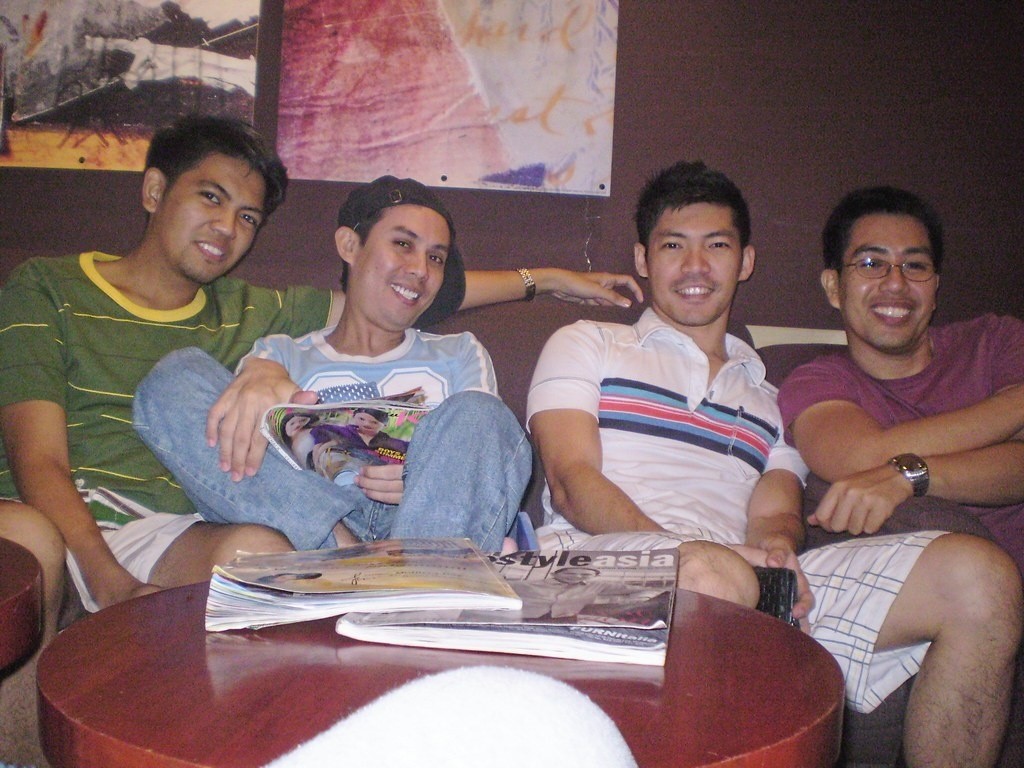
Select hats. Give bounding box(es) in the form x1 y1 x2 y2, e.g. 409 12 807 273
339 177 466 331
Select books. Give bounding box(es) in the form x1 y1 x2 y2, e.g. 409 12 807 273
259 385 437 486
203 535 681 667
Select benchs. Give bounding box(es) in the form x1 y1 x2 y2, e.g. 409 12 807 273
0 170 1024 768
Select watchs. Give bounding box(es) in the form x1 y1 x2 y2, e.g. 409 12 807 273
890 451 930 498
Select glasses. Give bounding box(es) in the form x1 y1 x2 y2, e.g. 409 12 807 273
840 258 941 282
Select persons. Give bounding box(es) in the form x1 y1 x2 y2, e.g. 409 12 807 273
775 185 1024 618
282 409 409 472
128 174 538 566
0 144 648 768
526 158 1024 767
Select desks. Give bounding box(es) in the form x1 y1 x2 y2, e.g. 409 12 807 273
35 576 843 768
0 536 41 668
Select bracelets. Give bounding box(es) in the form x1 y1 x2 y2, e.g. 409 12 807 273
517 264 539 302
